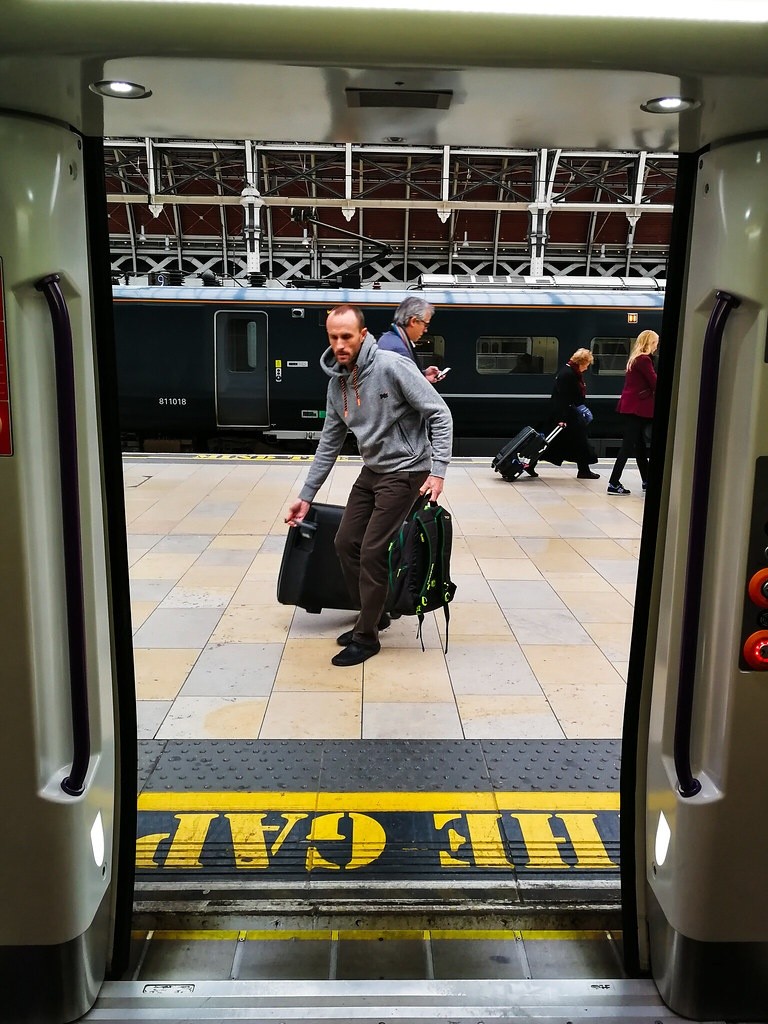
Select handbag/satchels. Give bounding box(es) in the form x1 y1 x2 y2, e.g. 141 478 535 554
575 404 593 425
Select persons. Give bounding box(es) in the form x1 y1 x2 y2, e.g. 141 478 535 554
377 296 448 384
605 329 660 496
524 347 601 480
285 303 455 668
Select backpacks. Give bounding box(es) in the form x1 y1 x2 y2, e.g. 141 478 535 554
387 494 456 620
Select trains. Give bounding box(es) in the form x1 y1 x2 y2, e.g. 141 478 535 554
113 269 669 454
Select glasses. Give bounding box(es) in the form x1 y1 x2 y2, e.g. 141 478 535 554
419 319 430 327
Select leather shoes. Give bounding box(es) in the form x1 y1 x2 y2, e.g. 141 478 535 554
331 631 382 668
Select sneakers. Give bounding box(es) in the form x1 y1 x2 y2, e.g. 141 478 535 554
608 484 631 495
642 483 647 491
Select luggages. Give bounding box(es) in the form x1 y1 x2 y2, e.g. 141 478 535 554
277 503 402 622
491 423 565 483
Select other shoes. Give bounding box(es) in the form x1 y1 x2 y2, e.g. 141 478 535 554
524 468 539 477
578 471 599 479
